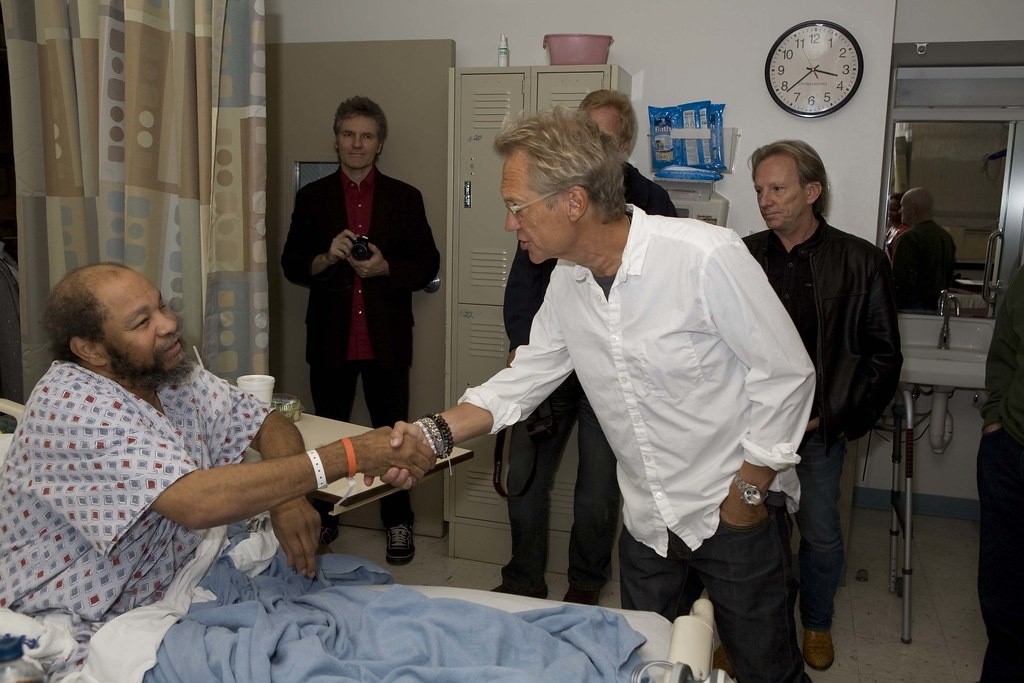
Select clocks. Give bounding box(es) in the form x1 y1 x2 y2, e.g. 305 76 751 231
765 19 864 119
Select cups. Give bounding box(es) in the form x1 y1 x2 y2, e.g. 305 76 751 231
236 374 275 407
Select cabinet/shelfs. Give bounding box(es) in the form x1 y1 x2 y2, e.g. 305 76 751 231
448 65 632 576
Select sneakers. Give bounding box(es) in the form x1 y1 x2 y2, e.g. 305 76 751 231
804 629 834 670
320 527 339 544
713 644 736 680
386 525 414 564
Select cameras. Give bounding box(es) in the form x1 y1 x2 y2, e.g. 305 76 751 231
350 235 373 261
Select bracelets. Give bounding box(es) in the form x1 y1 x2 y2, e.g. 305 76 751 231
341 437 357 477
306 449 327 489
413 413 454 459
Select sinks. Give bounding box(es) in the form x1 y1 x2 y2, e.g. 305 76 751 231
897 313 996 389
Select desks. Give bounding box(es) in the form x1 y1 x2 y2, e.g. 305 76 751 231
241 412 474 516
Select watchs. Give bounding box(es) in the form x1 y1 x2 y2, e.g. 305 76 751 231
734 473 768 505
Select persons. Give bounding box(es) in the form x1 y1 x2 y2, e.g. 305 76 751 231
885 193 910 262
363 103 817 683
975 263 1024 683
493 89 679 605
891 186 957 311
280 95 440 564
0 261 438 624
712 138 904 680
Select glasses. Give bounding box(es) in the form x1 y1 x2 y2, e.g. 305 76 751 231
505 191 559 214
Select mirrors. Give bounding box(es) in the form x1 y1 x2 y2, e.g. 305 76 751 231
883 120 1014 319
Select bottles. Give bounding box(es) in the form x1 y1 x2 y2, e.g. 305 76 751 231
498 33 508 67
0 638 48 683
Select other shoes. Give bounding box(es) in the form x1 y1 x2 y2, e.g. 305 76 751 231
563 586 600 606
489 583 549 599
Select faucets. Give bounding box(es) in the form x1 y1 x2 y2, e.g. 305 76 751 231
937 295 960 349
937 289 948 316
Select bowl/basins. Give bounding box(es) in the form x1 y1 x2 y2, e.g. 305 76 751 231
543 33 613 66
271 393 305 422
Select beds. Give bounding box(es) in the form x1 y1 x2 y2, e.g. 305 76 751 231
0 398 735 683
